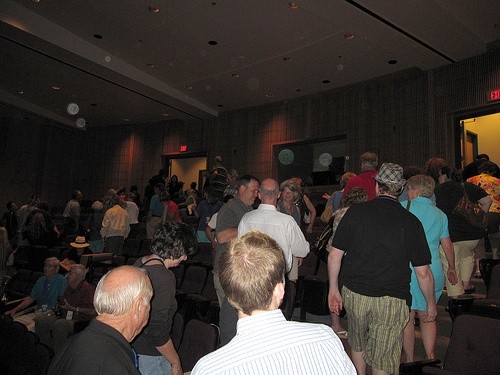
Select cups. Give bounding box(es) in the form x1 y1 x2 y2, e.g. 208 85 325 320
205 217 210 222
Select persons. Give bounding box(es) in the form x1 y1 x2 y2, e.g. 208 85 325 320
46 266 154 375
99 198 130 256
190 231 359 375
0 152 500 375
397 176 458 363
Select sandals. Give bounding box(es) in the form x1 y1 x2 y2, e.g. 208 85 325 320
337 330 348 338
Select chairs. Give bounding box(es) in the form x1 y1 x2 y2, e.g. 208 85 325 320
0 212 500 375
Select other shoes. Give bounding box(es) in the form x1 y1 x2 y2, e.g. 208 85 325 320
464 285 474 293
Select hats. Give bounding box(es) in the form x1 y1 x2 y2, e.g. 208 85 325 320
70 235 90 248
166 201 177 213
372 162 406 187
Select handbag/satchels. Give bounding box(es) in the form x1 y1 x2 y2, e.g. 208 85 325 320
453 194 487 231
312 215 335 264
